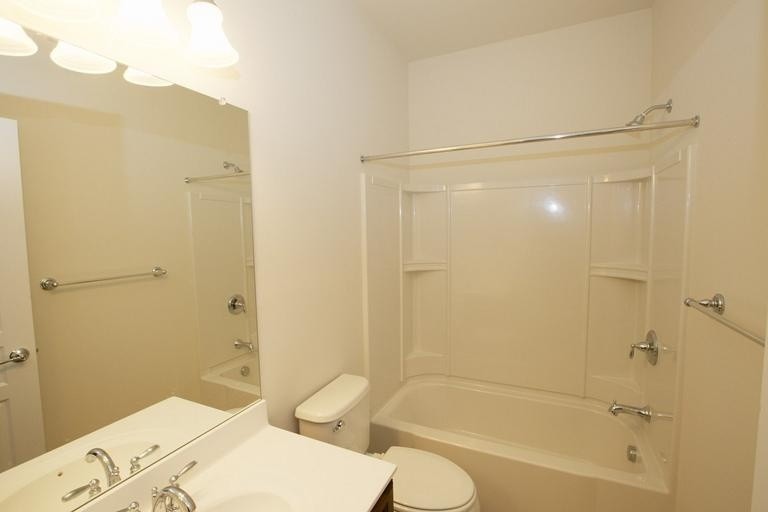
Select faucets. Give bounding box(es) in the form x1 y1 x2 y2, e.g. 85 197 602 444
234 339 253 352
609 402 650 423
150 460 200 512
84 445 120 487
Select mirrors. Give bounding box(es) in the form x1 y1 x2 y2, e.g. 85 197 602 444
0 17 263 512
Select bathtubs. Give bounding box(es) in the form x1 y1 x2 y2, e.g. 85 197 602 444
371 372 672 512
202 349 260 396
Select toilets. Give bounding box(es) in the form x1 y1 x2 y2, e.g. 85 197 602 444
294 373 482 512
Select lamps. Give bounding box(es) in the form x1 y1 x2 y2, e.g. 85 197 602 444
178 0 239 67
0 18 175 86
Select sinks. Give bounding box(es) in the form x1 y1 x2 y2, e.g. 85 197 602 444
0 435 162 512
197 490 294 512
